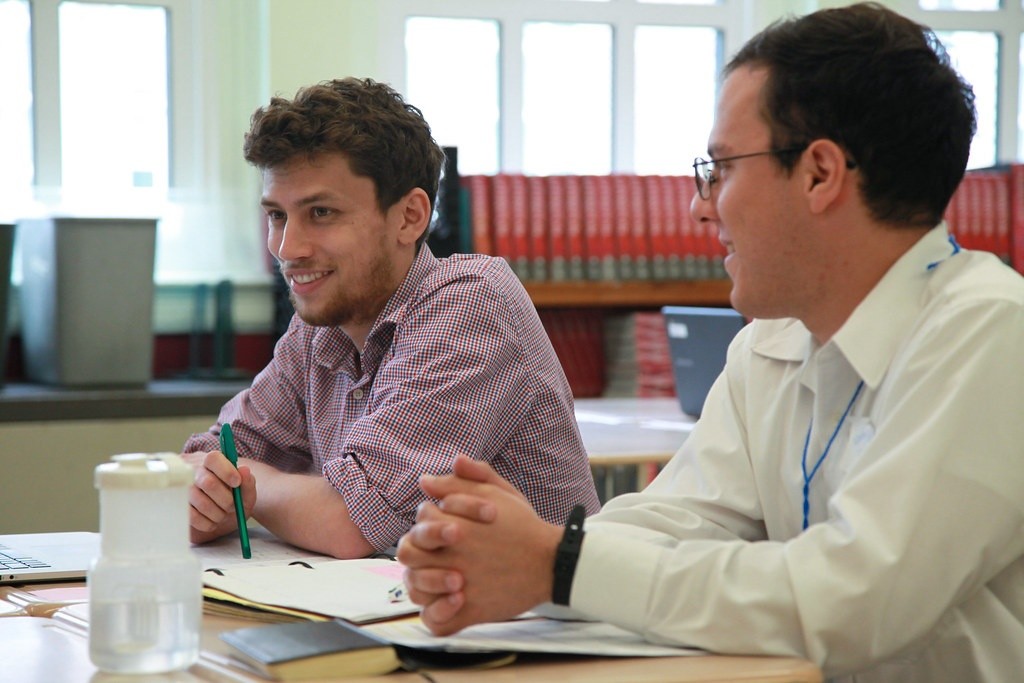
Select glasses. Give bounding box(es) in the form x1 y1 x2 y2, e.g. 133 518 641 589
692 145 857 200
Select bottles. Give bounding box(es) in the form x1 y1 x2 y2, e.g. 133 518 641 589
83 452 203 676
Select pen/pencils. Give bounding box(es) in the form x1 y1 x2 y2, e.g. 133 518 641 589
219 422 252 558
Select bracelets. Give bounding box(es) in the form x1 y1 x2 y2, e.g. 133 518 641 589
544 506 583 608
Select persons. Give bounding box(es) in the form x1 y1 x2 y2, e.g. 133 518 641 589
399 0 1024 683
173 80 596 554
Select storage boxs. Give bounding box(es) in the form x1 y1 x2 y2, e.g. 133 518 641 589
18 217 158 386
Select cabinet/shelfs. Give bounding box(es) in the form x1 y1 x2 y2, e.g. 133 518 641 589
0 376 252 534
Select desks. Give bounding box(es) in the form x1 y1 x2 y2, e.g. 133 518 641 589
574 397 704 502
0 580 826 683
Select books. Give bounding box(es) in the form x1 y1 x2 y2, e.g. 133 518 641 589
442 142 1023 305
